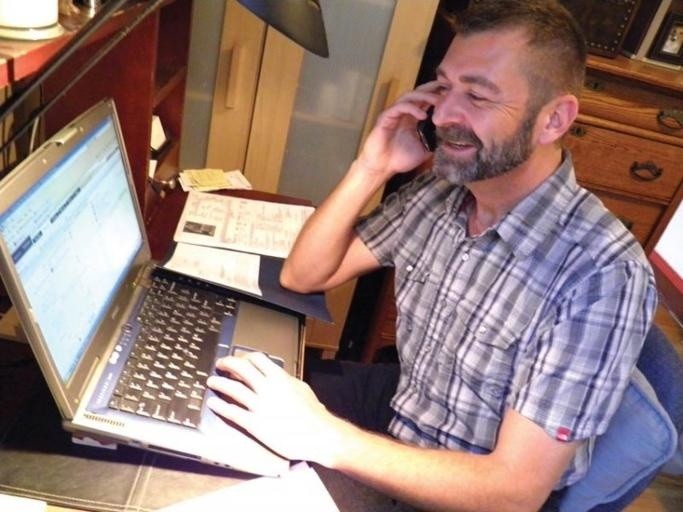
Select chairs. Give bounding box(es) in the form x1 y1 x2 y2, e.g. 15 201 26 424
635 322 683 475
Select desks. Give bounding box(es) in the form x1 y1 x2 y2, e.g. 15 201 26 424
0 184 319 512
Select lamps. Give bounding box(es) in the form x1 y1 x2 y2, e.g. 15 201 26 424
0 0 329 153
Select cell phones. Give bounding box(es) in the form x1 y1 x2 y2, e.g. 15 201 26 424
418 101 441 151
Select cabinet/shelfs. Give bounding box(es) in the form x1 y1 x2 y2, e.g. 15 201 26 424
363 8 683 361
0 1 192 226
181 1 439 360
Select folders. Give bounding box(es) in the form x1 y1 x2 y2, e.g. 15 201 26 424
155 191 336 324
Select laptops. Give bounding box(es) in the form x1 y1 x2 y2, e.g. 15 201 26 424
0 97 306 476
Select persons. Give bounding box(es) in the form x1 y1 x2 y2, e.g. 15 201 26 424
206 3 657 508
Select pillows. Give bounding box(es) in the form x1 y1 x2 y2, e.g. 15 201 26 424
557 367 679 512
